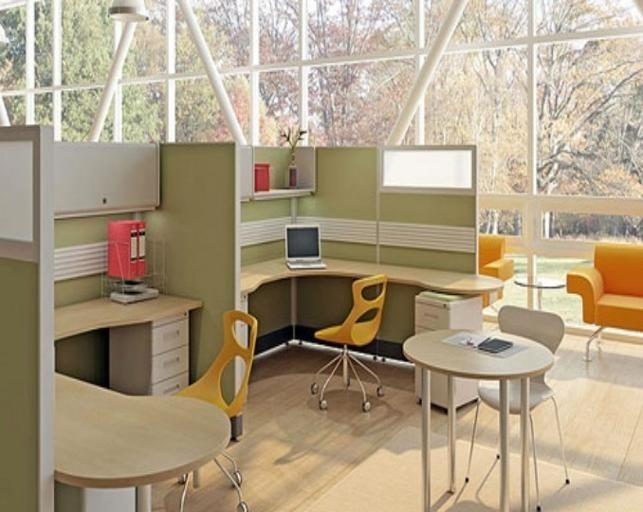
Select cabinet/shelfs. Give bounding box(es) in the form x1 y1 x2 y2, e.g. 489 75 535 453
412 289 483 412
110 311 190 397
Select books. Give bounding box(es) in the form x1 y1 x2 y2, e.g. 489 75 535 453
479 337 512 354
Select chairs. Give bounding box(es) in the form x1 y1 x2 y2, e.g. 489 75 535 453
465 306 569 512
310 275 387 411
172 310 259 511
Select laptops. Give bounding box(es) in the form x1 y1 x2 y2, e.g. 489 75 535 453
284 224 327 270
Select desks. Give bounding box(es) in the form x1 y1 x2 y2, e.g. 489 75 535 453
54 292 231 512
242 255 506 295
403 326 555 511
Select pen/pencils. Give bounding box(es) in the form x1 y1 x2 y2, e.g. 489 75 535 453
478 336 491 347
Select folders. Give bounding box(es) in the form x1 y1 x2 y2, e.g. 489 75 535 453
106 220 146 280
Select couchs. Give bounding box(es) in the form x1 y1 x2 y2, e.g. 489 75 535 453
566 242 643 362
478 232 514 314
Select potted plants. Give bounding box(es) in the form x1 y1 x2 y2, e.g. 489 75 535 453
275 122 307 187
